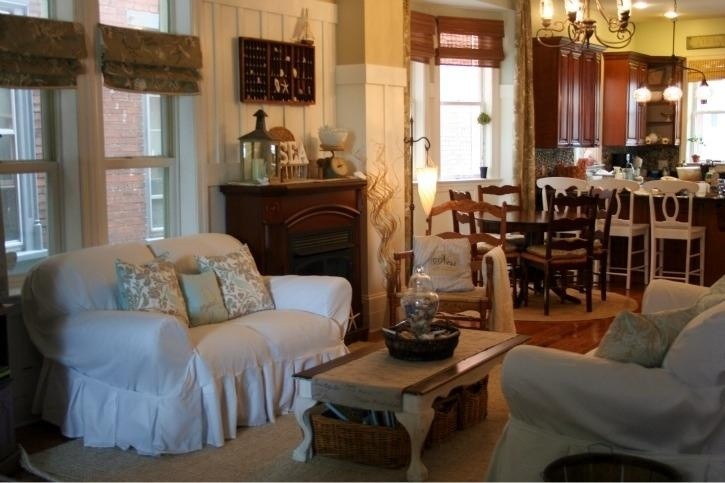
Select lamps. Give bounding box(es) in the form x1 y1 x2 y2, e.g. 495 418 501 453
536 0 636 51
633 0 711 105
403 116 437 251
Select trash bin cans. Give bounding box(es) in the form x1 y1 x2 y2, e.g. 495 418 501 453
541 450 683 483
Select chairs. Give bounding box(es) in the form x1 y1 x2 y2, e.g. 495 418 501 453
449 175 706 316
388 198 507 330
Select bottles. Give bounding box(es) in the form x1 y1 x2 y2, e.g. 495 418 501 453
401 266 439 333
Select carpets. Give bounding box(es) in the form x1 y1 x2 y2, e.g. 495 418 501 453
458 283 639 323
18 341 511 482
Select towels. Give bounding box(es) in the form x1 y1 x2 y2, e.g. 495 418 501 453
481 246 516 334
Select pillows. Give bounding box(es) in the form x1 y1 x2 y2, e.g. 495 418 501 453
111 248 190 329
192 243 275 320
176 268 230 327
594 304 699 367
410 234 473 291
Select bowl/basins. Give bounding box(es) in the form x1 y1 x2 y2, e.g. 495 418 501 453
319 129 347 146
383 322 461 360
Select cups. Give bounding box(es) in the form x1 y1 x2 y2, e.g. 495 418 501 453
695 181 710 198
648 131 662 143
648 91 662 102
662 137 670 144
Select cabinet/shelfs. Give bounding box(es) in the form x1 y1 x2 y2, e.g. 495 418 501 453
219 176 370 346
647 56 686 146
533 36 606 148
238 37 315 106
602 51 649 146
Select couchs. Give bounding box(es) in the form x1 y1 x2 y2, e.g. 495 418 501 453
19 232 354 458
477 276 725 482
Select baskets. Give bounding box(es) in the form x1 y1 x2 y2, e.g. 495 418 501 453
298 401 426 469
429 394 464 443
458 373 491 430
